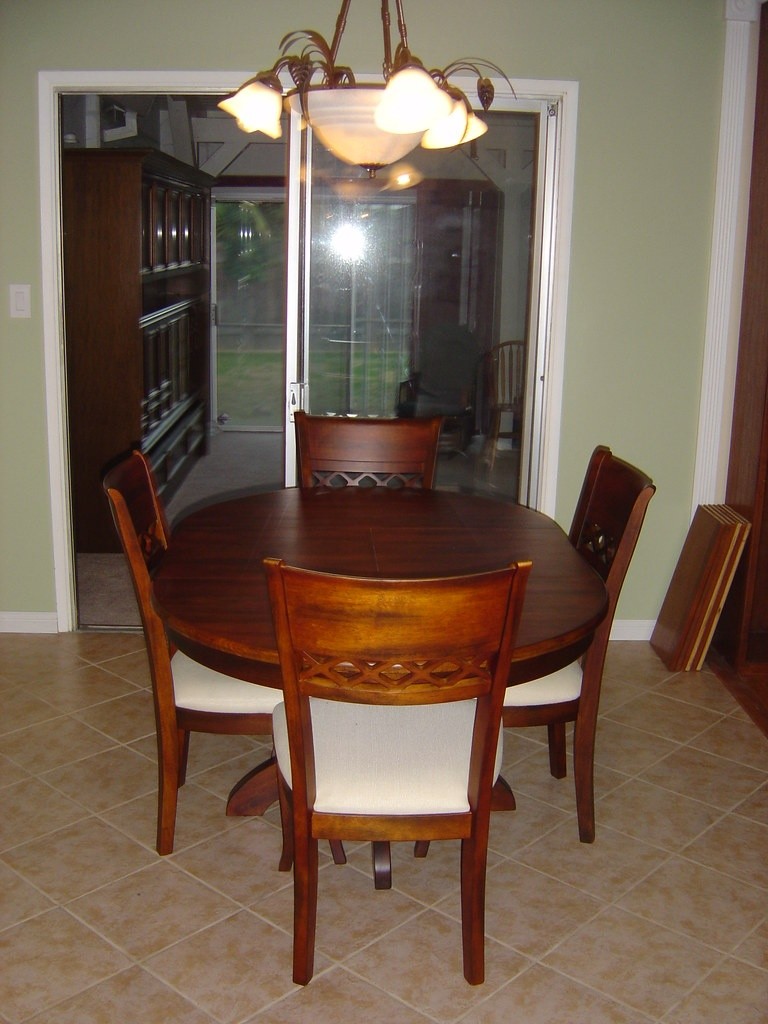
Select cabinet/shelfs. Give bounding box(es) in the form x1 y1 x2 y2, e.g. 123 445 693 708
61 145 211 558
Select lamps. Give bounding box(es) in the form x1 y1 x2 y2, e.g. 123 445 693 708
214 0 518 178
379 161 427 195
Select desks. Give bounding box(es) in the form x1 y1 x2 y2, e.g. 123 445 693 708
150 486 610 892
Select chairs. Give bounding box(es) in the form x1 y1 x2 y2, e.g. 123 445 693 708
293 407 444 492
99 448 348 867
262 554 531 990
412 442 657 861
469 338 524 471
